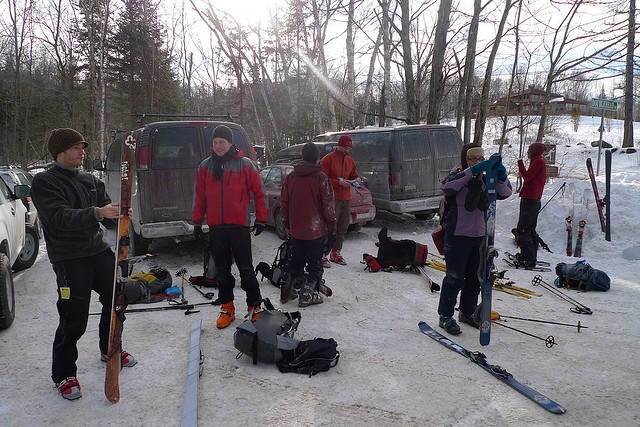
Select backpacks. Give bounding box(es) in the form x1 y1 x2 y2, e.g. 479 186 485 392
555 258 612 294
233 297 302 365
277 336 341 378
124 264 173 303
375 226 428 271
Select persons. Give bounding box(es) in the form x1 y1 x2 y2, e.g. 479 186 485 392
192 125 268 330
437 143 512 335
514 142 547 270
30 127 137 400
280 143 337 308
318 135 361 268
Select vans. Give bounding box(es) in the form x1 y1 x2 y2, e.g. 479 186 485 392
310 124 463 219
92 112 260 256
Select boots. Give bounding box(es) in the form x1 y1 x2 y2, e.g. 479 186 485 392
281 273 298 303
297 276 323 307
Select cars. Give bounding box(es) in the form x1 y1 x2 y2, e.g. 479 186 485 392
250 163 376 239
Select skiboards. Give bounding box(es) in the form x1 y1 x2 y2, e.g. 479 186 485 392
502 251 551 272
565 183 592 257
419 152 566 414
425 257 544 299
105 131 203 427
586 150 613 242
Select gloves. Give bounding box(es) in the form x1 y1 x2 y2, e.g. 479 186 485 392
252 221 265 235
323 232 336 255
477 189 489 210
465 180 482 211
193 223 204 240
470 159 493 172
495 161 506 179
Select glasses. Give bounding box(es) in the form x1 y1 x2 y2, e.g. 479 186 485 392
467 155 485 162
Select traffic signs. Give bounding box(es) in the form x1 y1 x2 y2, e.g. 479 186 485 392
592 97 618 111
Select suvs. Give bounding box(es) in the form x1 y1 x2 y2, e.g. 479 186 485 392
0 176 39 330
6 163 48 240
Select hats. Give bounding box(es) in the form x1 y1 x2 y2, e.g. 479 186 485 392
48 127 89 157
212 125 233 142
467 146 484 158
529 142 547 155
338 135 352 147
302 142 320 160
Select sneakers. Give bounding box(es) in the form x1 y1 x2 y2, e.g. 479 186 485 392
439 314 462 334
101 349 137 367
330 247 348 266
56 375 82 401
247 305 261 320
217 300 235 328
516 252 523 259
322 256 331 267
459 309 479 329
514 259 534 269
